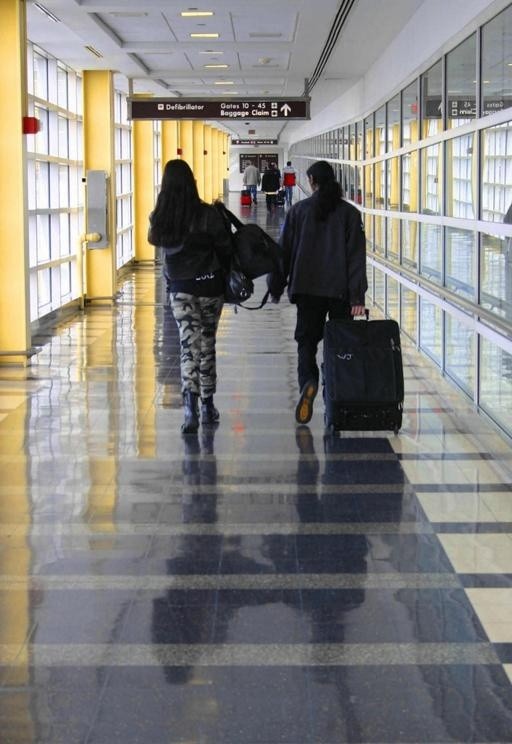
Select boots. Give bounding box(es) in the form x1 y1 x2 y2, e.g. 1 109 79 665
177 392 200 434
199 396 220 426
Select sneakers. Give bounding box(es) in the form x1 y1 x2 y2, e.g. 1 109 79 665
293 379 322 425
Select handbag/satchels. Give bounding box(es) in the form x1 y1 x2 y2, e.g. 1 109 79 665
222 253 256 305
211 197 283 281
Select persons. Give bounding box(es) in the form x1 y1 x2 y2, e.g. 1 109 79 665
241 160 262 206
150 419 221 688
280 426 373 686
265 158 367 424
260 161 296 216
147 159 234 434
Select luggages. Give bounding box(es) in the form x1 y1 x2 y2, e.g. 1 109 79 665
319 306 404 439
277 190 287 206
240 184 252 208
